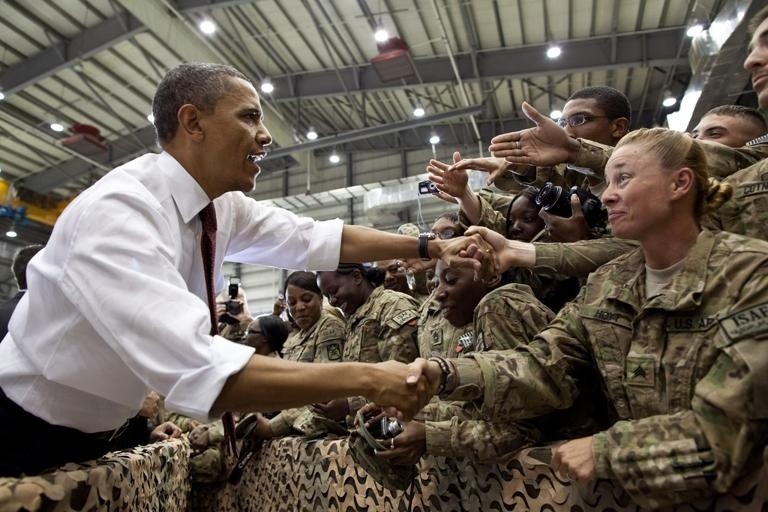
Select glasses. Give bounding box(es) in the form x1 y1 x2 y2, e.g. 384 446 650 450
243 329 260 335
428 228 456 240
557 113 606 129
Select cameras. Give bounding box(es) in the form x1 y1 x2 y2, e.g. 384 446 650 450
223 284 244 316
535 180 603 233
369 415 404 441
419 180 440 195
277 289 288 310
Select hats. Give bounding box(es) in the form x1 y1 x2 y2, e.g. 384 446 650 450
347 410 418 492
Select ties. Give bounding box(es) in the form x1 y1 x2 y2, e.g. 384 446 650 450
199 203 240 459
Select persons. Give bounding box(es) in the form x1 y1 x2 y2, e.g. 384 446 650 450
143 3 767 510
0 242 45 342
0 62 499 481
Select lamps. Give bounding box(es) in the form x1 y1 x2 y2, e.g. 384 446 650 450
200 9 215 33
685 10 703 37
545 39 561 58
307 118 318 140
260 28 275 94
328 146 340 163
49 86 64 132
413 101 425 117
661 87 675 109
429 126 440 144
373 1 388 42
550 98 562 120
5 223 17 238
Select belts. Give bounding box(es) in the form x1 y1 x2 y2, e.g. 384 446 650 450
80 421 129 444
